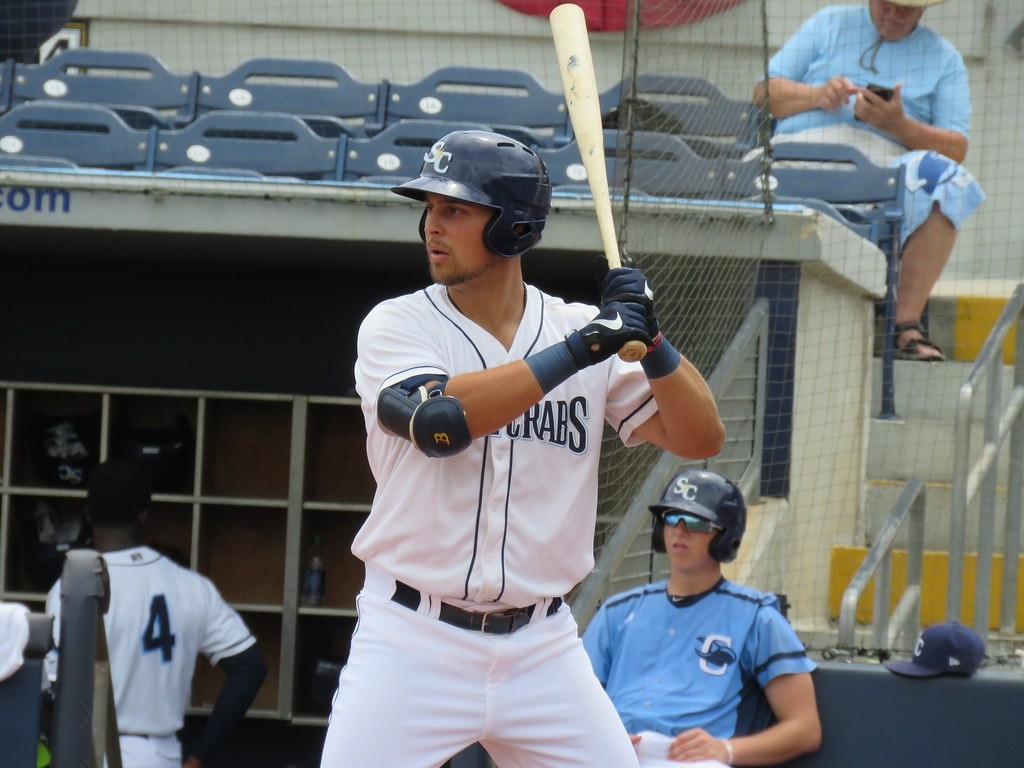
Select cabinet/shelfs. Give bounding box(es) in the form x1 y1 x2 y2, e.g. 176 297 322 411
0 378 377 729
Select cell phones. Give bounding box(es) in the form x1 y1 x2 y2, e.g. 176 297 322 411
855 83 893 121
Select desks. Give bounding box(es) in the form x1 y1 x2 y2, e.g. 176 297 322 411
745 662 1024 768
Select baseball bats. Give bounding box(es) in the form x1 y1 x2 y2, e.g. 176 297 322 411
548 3 647 363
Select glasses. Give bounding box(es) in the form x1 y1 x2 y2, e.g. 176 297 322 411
661 511 723 533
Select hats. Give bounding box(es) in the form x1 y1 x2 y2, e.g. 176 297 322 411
884 622 984 677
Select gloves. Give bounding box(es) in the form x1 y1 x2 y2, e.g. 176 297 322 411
580 302 653 362
598 267 660 352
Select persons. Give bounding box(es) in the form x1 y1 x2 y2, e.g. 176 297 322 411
319 129 726 768
41 458 270 768
581 469 822 768
754 0 985 362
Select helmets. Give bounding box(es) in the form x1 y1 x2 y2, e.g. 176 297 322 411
392 130 553 257
649 468 747 562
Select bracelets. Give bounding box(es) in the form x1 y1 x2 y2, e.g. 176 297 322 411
720 739 734 766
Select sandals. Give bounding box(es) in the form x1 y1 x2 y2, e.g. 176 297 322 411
894 320 945 362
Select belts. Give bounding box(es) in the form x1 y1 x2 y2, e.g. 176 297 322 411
391 580 560 634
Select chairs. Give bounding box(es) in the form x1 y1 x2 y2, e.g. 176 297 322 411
0 49 909 420
